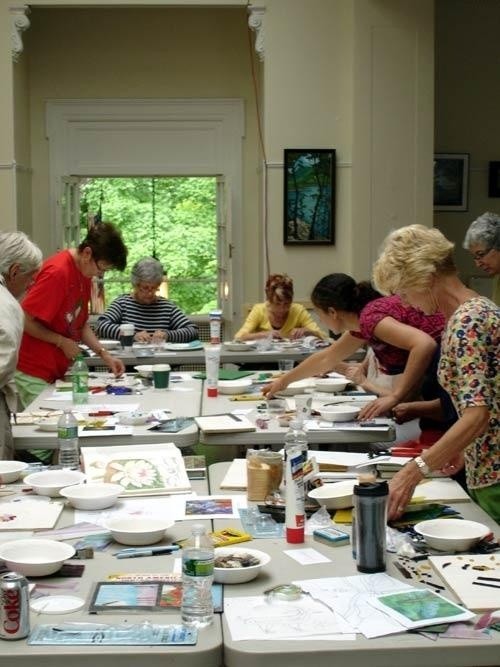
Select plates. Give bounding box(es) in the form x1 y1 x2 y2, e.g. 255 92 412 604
164 343 203 350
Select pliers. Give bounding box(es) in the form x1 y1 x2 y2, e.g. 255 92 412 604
89 410 116 416
373 447 424 457
89 386 105 394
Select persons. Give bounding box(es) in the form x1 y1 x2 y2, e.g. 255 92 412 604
462 210 500 308
233 273 458 432
14 222 127 410
0 230 42 461
93 256 199 346
372 224 500 524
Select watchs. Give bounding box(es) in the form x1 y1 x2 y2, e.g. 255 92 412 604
414 456 430 477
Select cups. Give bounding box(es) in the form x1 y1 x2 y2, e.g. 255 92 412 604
293 394 312 417
279 359 294 374
118 324 135 352
152 364 171 388
266 397 287 418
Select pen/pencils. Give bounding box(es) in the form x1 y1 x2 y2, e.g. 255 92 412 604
120 545 180 554
117 549 172 558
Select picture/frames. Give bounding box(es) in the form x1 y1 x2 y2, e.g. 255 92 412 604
283 148 335 244
434 152 471 213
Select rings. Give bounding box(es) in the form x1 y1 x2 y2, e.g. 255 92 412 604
397 507 404 512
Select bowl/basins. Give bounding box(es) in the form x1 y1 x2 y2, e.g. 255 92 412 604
223 341 255 350
308 479 360 510
34 420 57 431
103 513 175 545
22 470 86 497
315 406 361 422
311 378 351 392
98 341 120 350
116 412 149 425
133 365 154 377
414 518 489 551
218 378 252 394
213 547 271 584
0 461 29 484
59 483 122 509
0 539 76 577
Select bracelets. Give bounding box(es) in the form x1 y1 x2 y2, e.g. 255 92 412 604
97 348 105 354
56 336 62 349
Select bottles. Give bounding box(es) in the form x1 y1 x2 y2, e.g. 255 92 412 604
180 525 213 626
56 408 80 471
353 482 389 573
71 354 89 404
257 452 283 493
284 420 308 462
